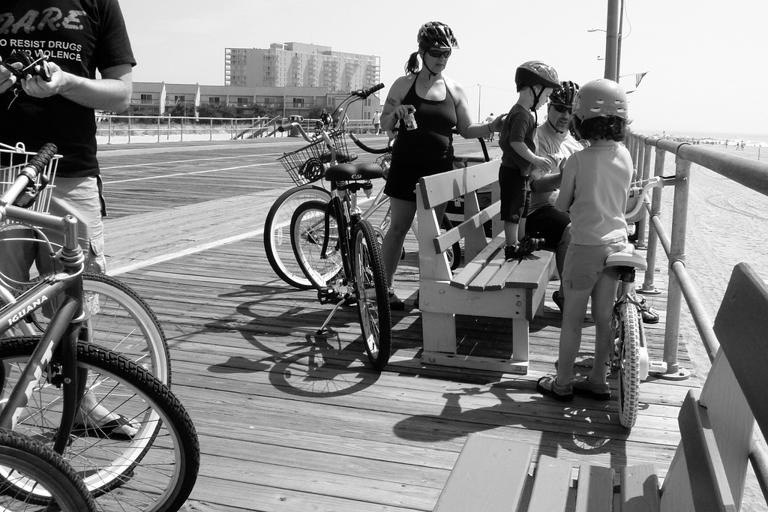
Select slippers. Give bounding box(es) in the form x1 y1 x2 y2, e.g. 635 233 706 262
74 414 134 439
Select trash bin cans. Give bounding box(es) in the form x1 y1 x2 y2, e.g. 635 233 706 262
289 115 304 137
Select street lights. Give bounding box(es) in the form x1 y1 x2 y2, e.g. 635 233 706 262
588 25 623 83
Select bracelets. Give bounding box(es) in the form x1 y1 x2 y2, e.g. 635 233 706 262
489 122 494 136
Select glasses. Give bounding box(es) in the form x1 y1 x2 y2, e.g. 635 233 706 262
428 49 451 57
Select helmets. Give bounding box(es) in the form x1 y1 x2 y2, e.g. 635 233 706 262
516 61 628 123
417 22 457 48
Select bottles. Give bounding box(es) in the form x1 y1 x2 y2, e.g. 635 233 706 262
530 153 559 181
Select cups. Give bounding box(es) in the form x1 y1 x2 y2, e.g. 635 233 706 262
403 110 418 132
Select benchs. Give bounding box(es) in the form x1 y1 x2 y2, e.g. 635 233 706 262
431 262 768 511
414 157 559 376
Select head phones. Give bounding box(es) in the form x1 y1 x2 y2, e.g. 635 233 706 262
0 49 51 82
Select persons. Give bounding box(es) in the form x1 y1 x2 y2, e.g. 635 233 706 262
483 112 496 141
525 80 660 326
536 77 636 401
334 108 350 136
370 109 382 134
500 61 561 260
319 106 333 132
380 23 511 310
0 0 141 441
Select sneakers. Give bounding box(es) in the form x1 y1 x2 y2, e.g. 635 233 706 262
505 236 545 258
537 377 610 401
